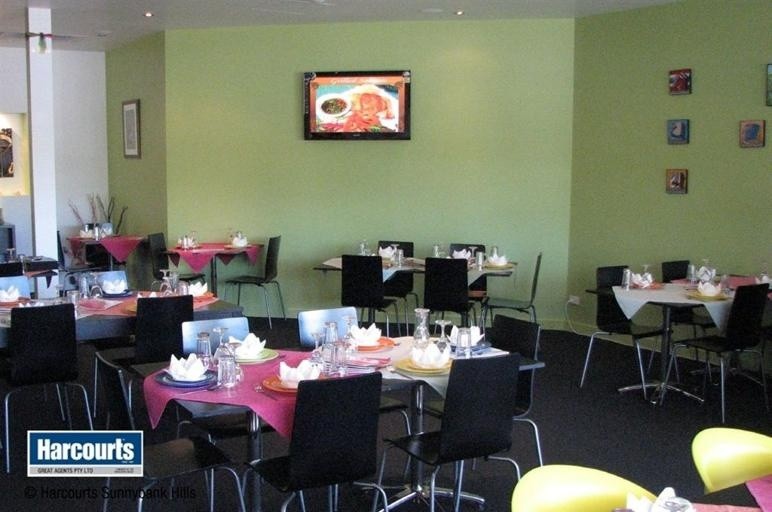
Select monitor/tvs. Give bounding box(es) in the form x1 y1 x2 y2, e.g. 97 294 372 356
303 70 411 140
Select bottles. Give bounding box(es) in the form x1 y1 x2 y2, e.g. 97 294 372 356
82 223 115 241
227 231 247 245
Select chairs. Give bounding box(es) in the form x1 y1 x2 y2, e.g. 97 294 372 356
0 223 772 512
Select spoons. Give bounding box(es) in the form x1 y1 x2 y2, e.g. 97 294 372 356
178 382 226 396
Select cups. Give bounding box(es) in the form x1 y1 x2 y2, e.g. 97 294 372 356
177 230 201 250
196 327 244 389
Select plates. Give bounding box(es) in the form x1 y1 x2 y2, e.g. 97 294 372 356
155 369 219 388
332 335 455 377
447 340 491 355
224 244 253 249
235 348 279 366
261 372 327 394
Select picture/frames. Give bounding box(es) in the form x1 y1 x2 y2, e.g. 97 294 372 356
122 99 141 158
0 113 31 199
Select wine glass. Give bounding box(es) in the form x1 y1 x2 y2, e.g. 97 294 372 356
620 257 772 302
307 314 358 377
412 305 472 359
67 270 189 310
359 238 509 269
